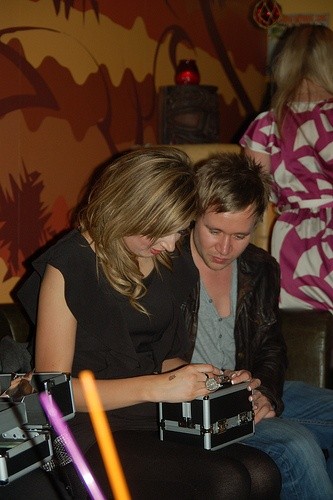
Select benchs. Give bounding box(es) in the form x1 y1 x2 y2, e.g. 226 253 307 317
0 303 332 498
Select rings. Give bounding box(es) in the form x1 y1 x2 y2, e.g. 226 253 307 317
204 367 218 391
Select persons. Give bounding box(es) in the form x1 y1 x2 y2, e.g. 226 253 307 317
184 152 333 500
17 146 284 500
240 24 333 316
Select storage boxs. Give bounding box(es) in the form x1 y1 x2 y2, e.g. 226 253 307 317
0 371 76 484
157 381 255 450
